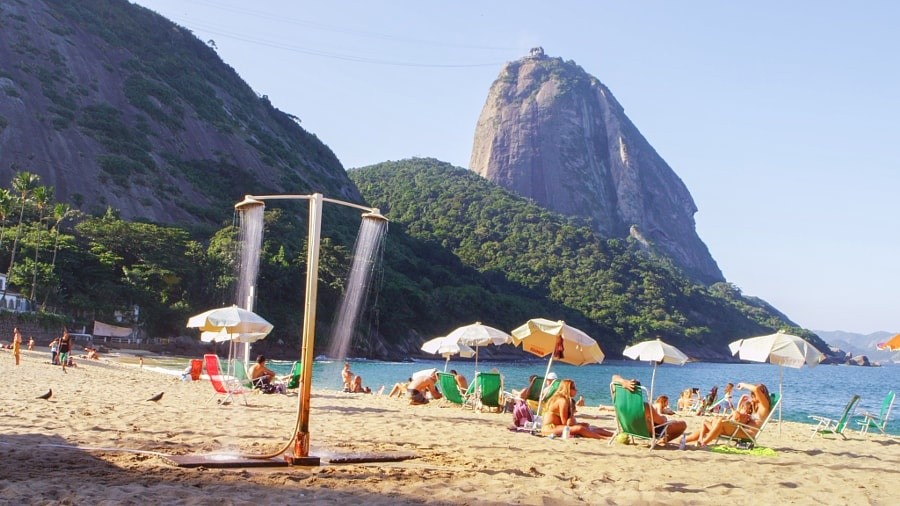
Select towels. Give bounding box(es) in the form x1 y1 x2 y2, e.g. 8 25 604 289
553 334 565 359
703 443 777 456
507 398 532 431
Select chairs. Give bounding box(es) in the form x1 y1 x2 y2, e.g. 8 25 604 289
434 371 895 450
178 353 301 408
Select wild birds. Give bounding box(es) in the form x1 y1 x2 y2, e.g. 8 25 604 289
35 388 52 401
147 392 164 403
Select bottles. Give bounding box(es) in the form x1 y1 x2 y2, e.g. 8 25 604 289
563 426 570 440
679 435 686 450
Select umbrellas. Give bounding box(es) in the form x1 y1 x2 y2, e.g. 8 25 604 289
622 338 688 402
420 336 477 373
446 321 515 372
186 304 275 380
728 329 827 440
511 318 606 428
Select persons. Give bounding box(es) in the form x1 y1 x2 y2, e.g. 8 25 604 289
248 355 275 388
611 375 686 445
389 378 412 398
26 336 34 350
140 358 143 367
342 363 353 392
512 373 560 399
677 383 771 448
542 379 617 441
406 373 442 405
450 370 467 394
49 337 77 367
351 376 371 392
13 328 22 365
87 347 99 360
57 330 72 374
